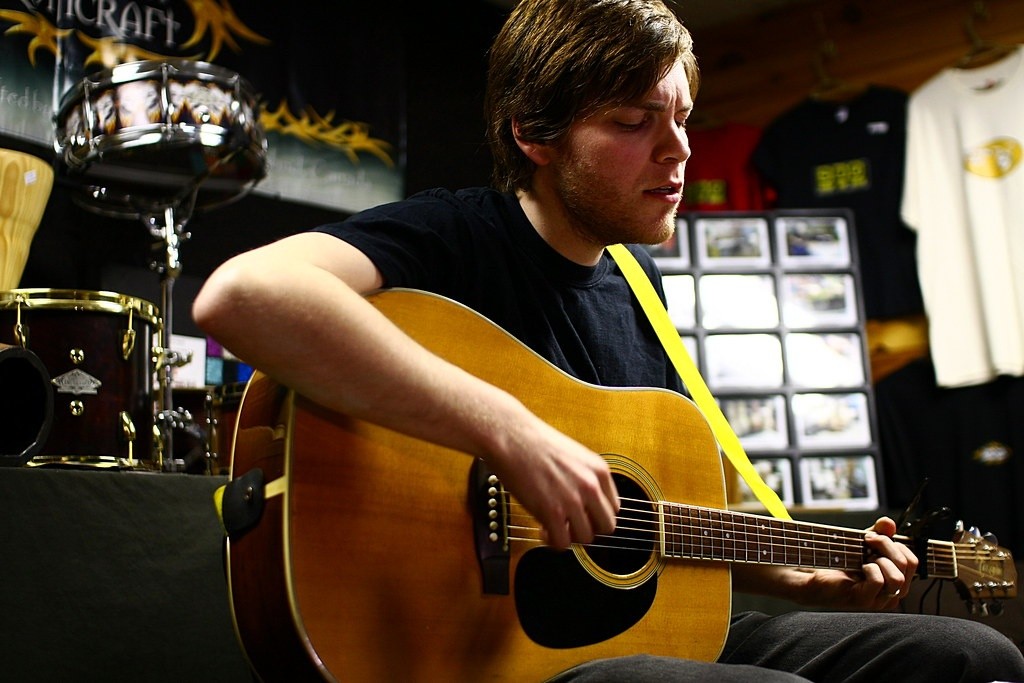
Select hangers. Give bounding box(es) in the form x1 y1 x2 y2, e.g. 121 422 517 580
811 42 876 101
951 10 1022 71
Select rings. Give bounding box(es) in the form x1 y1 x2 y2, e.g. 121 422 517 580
878 589 901 604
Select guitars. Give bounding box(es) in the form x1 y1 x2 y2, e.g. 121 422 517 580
218 282 1024 683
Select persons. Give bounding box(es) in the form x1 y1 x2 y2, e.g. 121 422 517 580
192 0 1024 683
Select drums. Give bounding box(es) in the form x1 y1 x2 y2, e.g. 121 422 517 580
0 284 168 475
203 380 249 477
49 55 273 225
